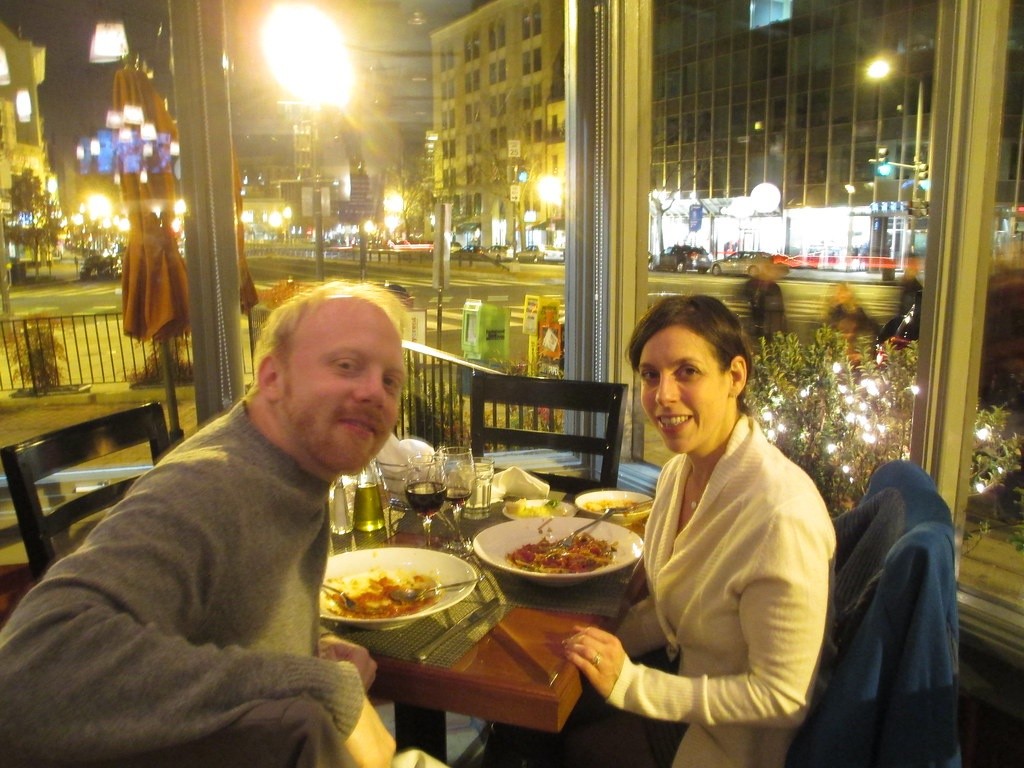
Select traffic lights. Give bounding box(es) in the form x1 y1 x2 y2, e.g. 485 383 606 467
920 201 928 217
919 163 929 190
908 199 920 217
878 147 890 175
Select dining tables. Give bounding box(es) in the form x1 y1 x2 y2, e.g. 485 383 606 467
319 467 647 765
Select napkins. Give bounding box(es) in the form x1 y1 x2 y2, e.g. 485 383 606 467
375 432 434 472
487 466 550 507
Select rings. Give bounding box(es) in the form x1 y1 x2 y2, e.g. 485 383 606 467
592 651 602 666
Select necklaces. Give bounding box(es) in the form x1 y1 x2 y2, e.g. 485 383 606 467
681 486 706 511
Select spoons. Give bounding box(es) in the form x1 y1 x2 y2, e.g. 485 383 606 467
389 575 485 602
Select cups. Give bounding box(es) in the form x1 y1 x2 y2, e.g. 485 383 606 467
463 457 494 520
329 454 385 535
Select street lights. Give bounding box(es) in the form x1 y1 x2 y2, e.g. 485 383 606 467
866 61 925 261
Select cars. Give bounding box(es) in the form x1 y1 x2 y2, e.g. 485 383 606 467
452 245 485 255
79 254 122 280
515 243 564 264
711 250 788 280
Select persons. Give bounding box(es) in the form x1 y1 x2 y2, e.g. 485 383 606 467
894 252 925 345
826 283 874 370
733 261 786 341
0 284 403 768
484 295 837 768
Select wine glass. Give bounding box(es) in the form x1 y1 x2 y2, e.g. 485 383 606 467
405 446 474 557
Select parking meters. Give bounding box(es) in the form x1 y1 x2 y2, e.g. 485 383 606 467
75 256 79 276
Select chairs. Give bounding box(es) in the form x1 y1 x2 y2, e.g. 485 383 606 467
0 367 957 768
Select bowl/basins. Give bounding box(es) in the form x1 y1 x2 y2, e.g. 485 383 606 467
319 548 477 631
473 517 643 588
576 490 654 526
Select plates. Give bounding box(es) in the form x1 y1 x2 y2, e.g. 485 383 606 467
502 499 576 520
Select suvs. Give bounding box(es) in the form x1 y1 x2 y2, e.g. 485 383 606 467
482 246 514 262
648 245 710 273
450 241 462 254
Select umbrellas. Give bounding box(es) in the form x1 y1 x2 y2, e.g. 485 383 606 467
238 146 258 317
111 65 192 344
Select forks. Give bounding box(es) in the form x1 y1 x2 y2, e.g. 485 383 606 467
545 508 617 556
321 584 385 614
